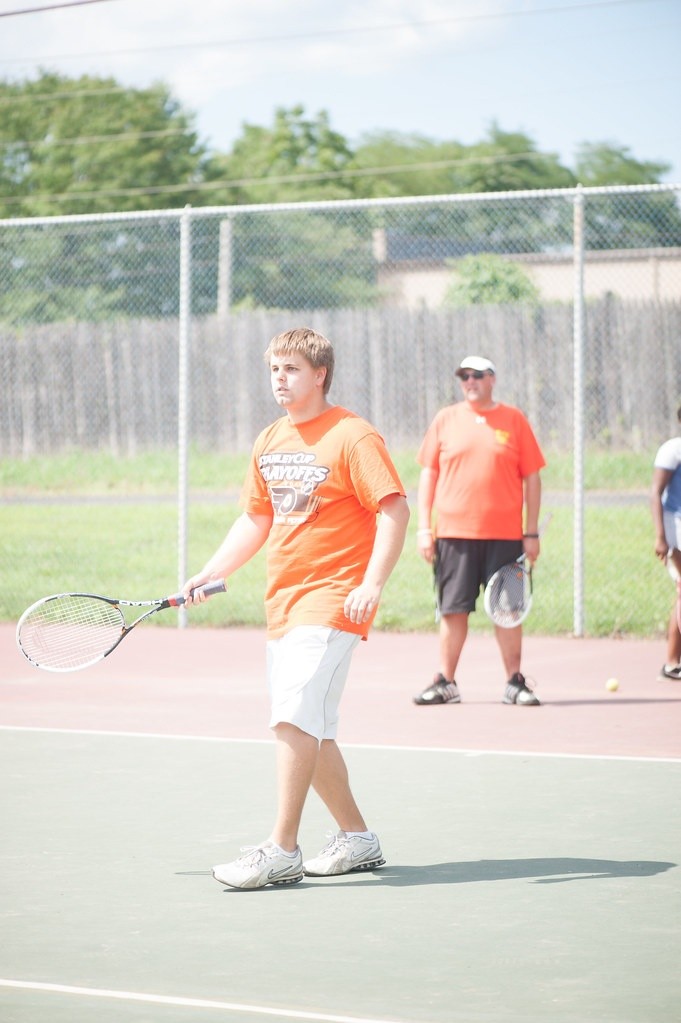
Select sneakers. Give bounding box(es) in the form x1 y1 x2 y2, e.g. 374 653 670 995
415 673 461 705
304 829 386 877
502 672 540 705
211 838 304 889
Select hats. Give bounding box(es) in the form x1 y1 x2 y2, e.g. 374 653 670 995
456 356 496 374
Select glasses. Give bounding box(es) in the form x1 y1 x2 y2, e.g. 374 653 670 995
460 371 493 381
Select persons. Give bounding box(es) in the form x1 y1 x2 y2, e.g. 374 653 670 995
413 356 544 707
183 328 409 889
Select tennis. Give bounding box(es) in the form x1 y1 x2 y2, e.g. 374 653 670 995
605 678 619 692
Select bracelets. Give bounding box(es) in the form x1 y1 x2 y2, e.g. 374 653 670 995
524 534 538 538
420 529 432 536
651 407 681 681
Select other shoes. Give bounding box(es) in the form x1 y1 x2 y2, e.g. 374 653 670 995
661 663 681 679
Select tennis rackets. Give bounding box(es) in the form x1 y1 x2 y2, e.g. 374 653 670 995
14 577 229 675
482 552 535 630
